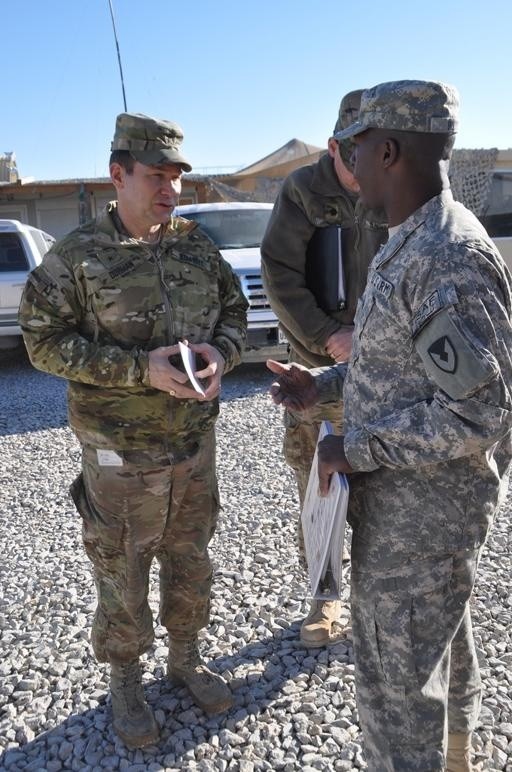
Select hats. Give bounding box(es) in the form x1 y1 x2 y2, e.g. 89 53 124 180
334 79 461 174
110 113 192 172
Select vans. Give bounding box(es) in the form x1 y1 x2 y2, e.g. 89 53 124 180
171 201 292 364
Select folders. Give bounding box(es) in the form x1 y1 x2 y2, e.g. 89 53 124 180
307 224 351 311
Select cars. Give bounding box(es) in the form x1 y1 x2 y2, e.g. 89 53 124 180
1 218 56 349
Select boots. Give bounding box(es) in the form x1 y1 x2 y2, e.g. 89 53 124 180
300 600 341 648
167 639 233 714
445 734 472 772
110 662 160 747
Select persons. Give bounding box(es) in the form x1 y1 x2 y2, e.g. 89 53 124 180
259 87 389 651
17 110 253 753
262 72 512 771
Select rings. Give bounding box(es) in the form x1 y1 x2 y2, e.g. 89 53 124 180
168 390 177 397
331 352 338 360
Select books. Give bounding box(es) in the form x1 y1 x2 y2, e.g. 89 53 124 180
177 339 207 399
